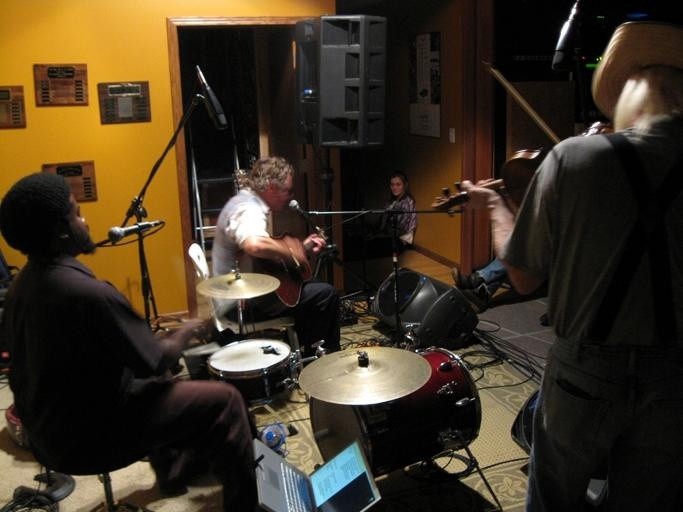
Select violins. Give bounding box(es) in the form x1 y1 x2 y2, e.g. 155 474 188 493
431 120 614 218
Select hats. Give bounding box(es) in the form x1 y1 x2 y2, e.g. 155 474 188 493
590 20 683 120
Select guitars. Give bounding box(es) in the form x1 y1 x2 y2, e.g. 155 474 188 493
240 227 328 318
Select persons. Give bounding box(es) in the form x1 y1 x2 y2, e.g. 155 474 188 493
360 172 418 296
449 255 506 307
458 19 681 512
213 155 340 365
1 172 264 512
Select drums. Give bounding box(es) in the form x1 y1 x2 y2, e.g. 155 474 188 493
311 347 482 479
205 338 293 401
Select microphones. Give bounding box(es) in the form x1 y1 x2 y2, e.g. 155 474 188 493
195 64 229 129
288 199 319 233
108 219 165 242
550 0 586 72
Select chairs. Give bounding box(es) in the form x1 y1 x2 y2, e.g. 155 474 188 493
187 241 304 370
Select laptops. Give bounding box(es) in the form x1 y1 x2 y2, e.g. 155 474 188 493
252 436 382 512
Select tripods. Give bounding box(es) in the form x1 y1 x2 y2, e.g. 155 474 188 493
380 214 413 348
315 148 377 298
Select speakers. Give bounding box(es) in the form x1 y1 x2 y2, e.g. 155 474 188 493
293 13 387 146
369 265 480 350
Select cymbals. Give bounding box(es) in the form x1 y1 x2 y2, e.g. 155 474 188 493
196 273 280 299
298 348 432 405
